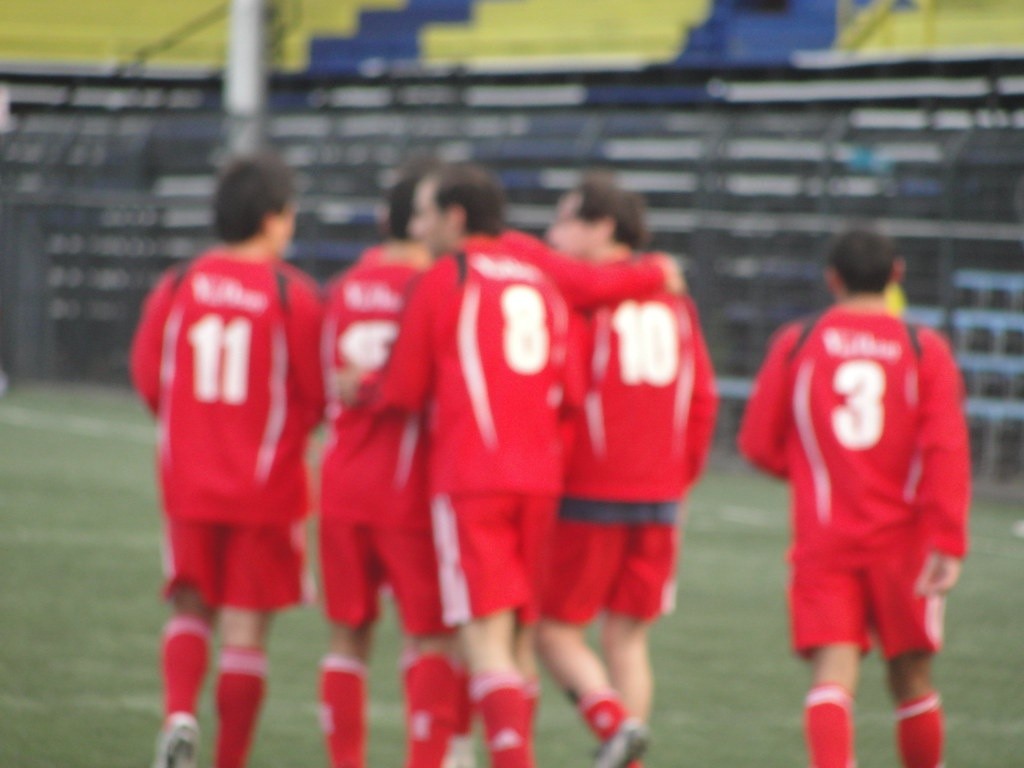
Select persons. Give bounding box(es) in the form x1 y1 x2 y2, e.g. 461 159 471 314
530 179 719 767
736 227 972 768
314 176 491 768
329 161 690 768
127 164 341 768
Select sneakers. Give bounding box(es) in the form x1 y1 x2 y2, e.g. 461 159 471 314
592 713 651 768
156 709 198 768
